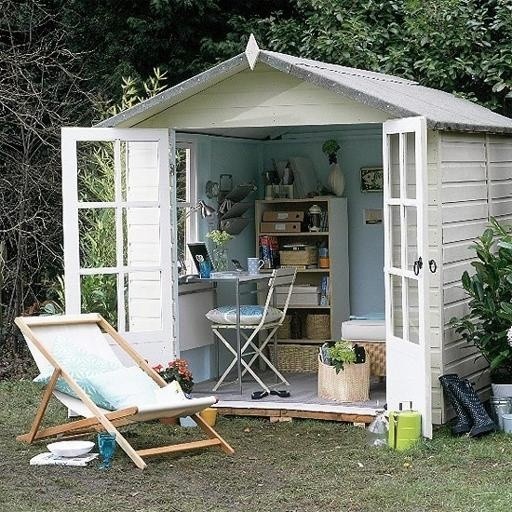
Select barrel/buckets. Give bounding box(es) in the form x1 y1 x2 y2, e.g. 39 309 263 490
490 396 512 430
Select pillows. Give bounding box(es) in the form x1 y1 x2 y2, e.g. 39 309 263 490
204 305 284 324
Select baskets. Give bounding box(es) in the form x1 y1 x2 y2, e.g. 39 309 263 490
267 344 320 374
269 315 293 339
306 314 331 340
317 342 371 403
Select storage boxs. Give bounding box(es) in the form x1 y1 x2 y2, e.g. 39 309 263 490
275 285 321 307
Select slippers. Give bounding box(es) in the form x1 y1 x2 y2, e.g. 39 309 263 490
270 387 290 397
251 390 269 399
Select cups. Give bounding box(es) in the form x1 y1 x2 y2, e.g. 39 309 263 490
200 261 210 277
247 257 264 275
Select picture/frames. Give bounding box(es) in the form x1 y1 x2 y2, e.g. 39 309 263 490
360 167 383 226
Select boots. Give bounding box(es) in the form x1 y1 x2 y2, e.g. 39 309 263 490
448 378 498 439
439 374 474 435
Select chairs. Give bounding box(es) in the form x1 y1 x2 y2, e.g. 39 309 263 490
13 313 235 469
205 265 298 394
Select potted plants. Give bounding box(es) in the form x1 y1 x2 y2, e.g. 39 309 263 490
450 215 512 434
322 139 346 197
206 228 236 271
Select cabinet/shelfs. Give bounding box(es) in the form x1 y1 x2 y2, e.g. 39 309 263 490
255 197 350 372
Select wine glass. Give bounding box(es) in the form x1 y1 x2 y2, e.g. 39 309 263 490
97 433 117 469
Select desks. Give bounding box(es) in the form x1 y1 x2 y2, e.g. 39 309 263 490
180 273 278 395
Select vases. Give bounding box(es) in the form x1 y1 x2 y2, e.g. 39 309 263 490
502 413 512 433
158 406 218 427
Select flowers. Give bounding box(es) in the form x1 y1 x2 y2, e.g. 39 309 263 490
154 361 194 394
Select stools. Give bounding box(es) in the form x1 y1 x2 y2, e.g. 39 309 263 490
342 308 386 401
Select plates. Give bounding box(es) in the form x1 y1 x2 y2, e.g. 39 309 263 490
45 440 95 458
